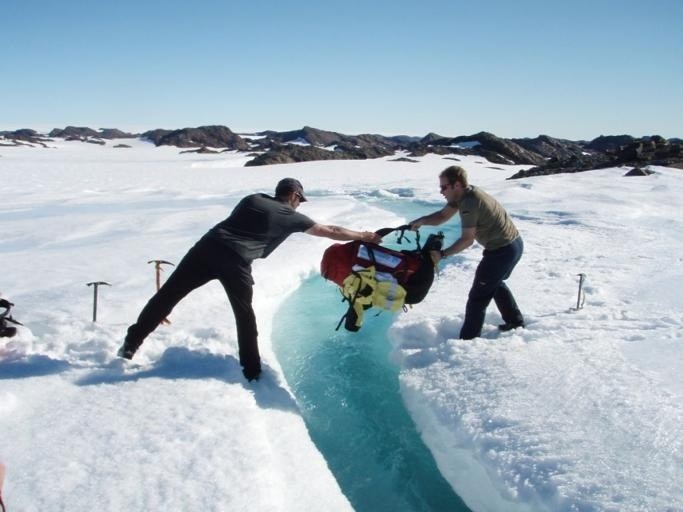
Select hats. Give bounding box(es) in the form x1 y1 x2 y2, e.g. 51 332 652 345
275 177 308 203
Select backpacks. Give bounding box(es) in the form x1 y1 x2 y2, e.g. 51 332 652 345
320 224 447 305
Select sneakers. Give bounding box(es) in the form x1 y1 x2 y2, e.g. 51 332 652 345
498 322 526 331
117 343 137 360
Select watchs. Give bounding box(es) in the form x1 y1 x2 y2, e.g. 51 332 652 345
441 250 446 259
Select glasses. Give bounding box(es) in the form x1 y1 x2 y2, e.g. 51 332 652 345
440 182 455 191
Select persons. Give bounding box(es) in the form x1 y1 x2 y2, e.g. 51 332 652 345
410 166 524 339
117 179 383 382
